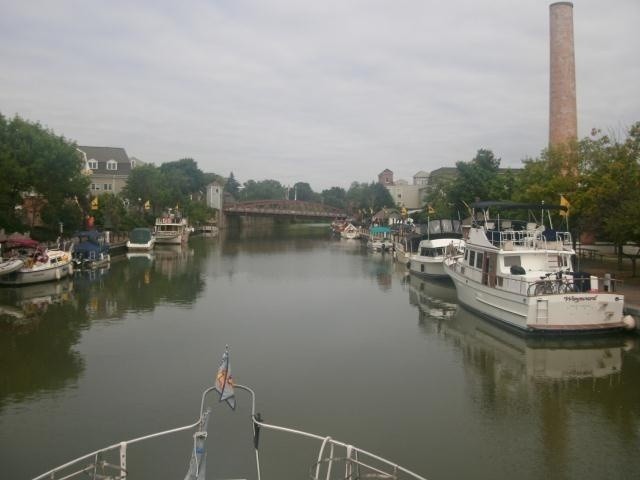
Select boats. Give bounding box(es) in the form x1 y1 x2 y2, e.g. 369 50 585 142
408 273 459 324
199 219 218 232
330 218 465 275
126 208 194 250
443 201 628 331
0 231 111 285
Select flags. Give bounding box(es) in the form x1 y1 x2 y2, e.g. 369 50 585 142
560 196 570 217
92 197 98 210
215 350 236 410
428 204 436 214
